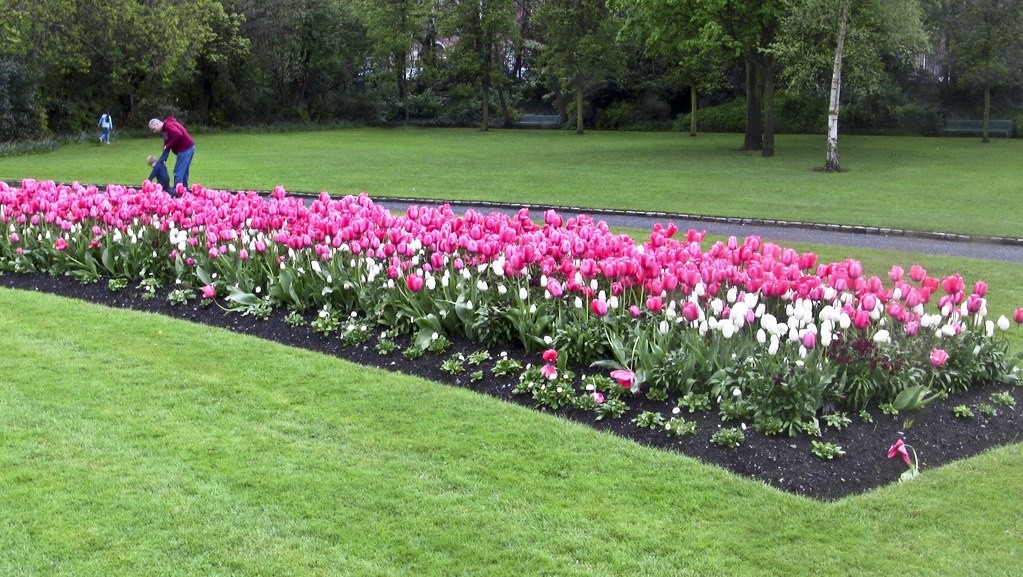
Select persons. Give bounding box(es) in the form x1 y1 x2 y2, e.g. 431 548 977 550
98 110 112 144
147 148 172 195
148 116 194 195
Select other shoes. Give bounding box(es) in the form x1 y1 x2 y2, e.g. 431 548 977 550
105 142 110 144
98 137 102 143
